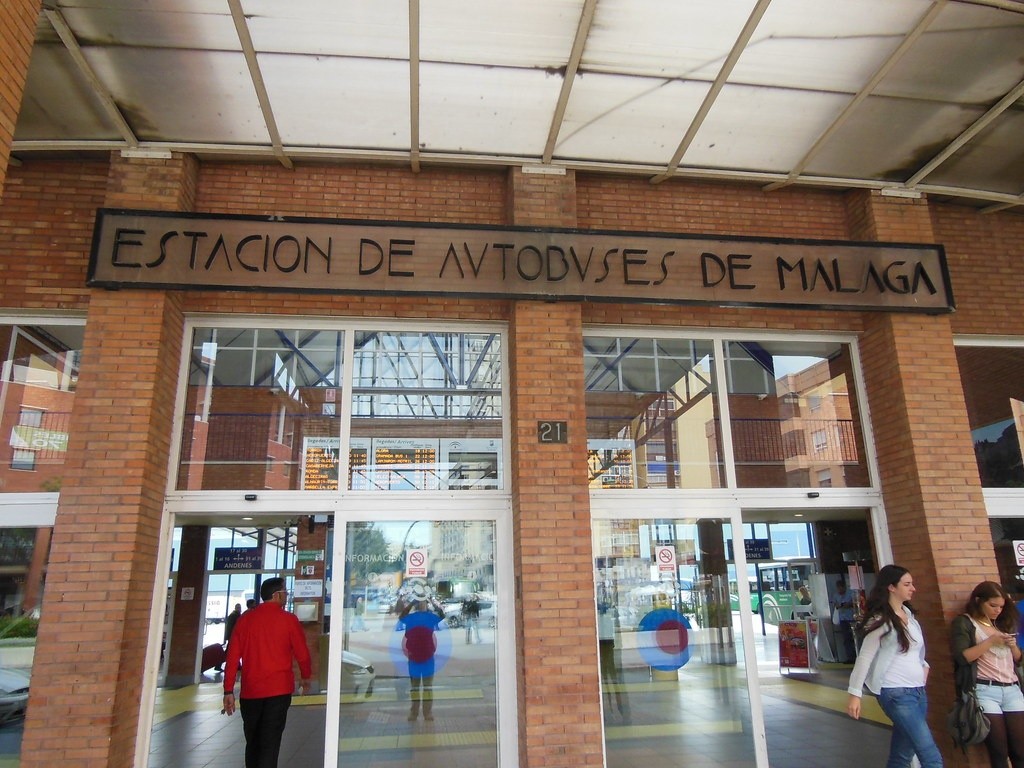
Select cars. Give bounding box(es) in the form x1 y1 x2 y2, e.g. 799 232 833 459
444 601 497 629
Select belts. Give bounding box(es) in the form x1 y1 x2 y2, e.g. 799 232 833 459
975 679 1018 686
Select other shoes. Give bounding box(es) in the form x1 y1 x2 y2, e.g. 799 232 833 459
423 710 434 721
408 711 418 721
214 667 223 671
843 658 855 664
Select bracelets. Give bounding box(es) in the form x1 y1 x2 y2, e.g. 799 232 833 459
224 691 234 695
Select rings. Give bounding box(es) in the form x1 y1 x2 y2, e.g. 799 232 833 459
304 688 308 690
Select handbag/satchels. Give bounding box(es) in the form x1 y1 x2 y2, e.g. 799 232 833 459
833 605 841 625
946 693 991 747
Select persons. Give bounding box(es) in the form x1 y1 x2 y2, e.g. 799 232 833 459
596 583 633 725
659 593 666 609
949 581 1024 768
791 587 817 640
848 565 943 768
389 593 445 721
462 594 481 644
834 579 861 664
215 577 312 768
351 596 369 632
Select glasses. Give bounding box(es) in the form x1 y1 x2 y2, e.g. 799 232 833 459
271 588 289 596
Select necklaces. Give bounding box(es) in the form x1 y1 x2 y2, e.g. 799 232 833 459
976 619 994 626
893 606 909 625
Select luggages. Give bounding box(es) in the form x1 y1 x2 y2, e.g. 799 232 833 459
201 643 227 673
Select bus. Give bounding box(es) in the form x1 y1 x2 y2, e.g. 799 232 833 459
729 577 764 613
757 557 820 625
